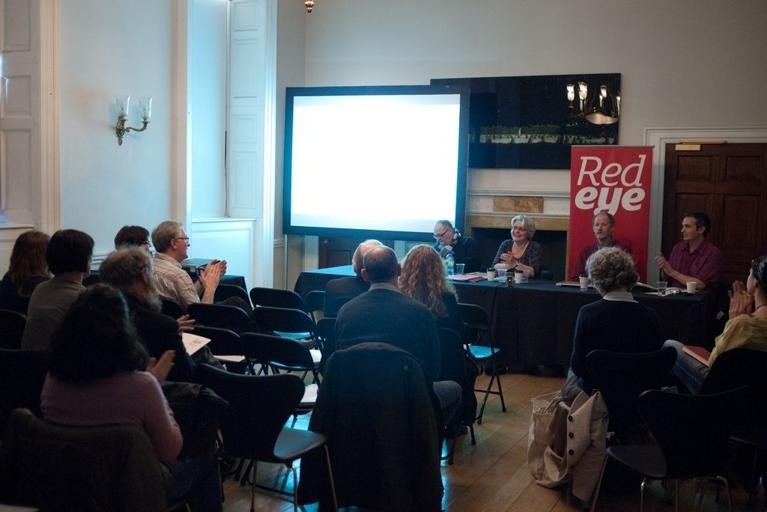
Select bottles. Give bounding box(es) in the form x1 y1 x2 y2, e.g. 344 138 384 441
445 250 454 275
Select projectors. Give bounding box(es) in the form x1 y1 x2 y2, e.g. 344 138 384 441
180 258 213 277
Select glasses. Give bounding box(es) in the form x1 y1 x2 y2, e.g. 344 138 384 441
434 229 449 238
169 237 189 243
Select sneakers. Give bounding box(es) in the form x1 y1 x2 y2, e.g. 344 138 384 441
445 424 468 439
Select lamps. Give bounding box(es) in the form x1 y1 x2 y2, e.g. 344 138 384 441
115 95 151 146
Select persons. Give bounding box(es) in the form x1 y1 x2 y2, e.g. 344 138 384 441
567 246 662 395
2 220 227 512
431 220 481 272
661 255 767 395
655 212 726 289
321 238 478 440
491 215 541 279
573 210 630 282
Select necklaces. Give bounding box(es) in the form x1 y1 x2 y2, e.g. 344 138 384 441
757 304 767 309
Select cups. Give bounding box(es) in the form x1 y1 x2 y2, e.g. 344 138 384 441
656 281 667 296
456 263 465 275
486 265 529 286
685 281 697 294
578 273 588 288
